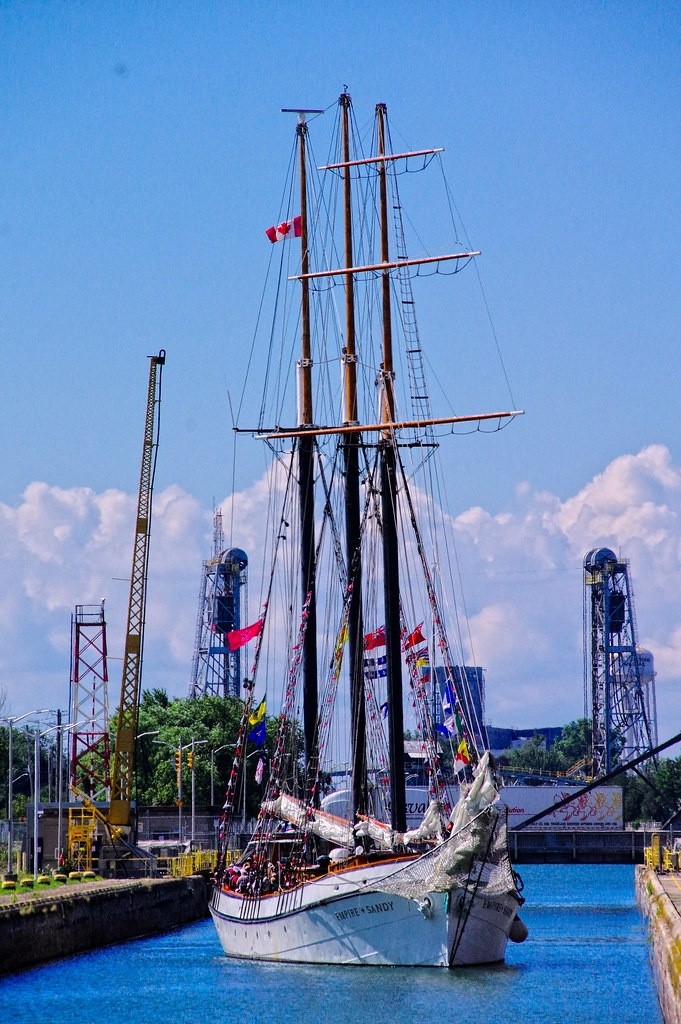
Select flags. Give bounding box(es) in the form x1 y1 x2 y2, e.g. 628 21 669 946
248 694 266 746
364 625 386 650
406 646 429 665
364 655 386 679
454 739 469 774
254 755 266 785
437 715 457 739
227 619 262 652
401 621 427 652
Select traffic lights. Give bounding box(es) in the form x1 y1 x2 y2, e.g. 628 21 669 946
175 750 180 773
187 752 193 769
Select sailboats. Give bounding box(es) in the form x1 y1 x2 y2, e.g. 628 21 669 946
208 85 526 969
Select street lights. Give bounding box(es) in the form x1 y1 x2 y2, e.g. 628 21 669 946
19 724 71 881
0 709 50 881
244 750 263 826
211 744 237 806
27 720 96 868
152 740 208 844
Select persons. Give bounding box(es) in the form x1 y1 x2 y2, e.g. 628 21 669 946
59 852 64 866
210 855 284 896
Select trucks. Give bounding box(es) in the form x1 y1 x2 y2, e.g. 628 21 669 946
321 786 624 831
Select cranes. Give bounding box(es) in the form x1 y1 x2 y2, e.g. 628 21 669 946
65 349 160 876
188 497 248 700
